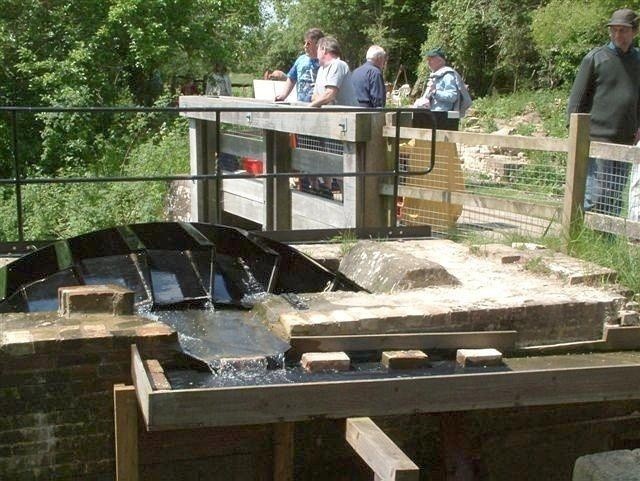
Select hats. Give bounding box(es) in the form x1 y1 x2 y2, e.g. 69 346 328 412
604 9 640 28
427 48 446 59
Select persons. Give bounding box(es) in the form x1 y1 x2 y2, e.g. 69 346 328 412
353 44 386 106
181 62 234 96
564 8 640 244
274 27 339 194
307 34 350 203
421 49 473 121
264 67 286 82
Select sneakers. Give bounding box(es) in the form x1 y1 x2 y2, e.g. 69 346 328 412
294 180 341 200
